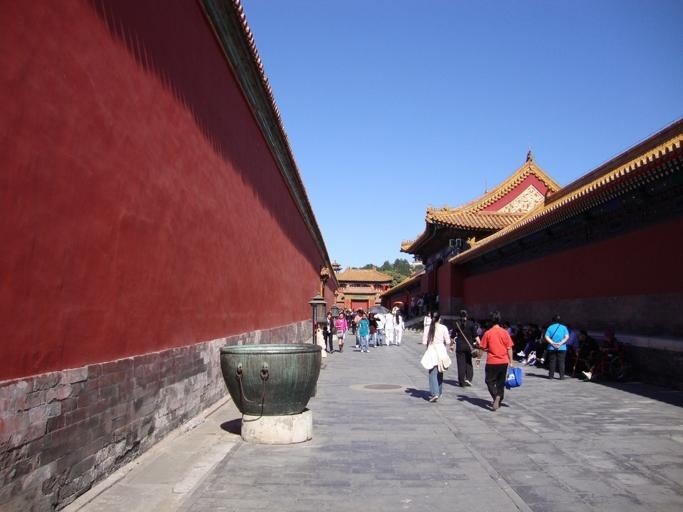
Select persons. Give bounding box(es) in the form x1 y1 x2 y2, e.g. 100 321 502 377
418 312 452 403
475 310 514 411
334 313 348 353
391 288 439 321
473 319 546 367
422 311 432 345
341 307 394 348
320 312 337 354
392 310 406 346
581 324 619 382
450 308 481 387
544 314 570 379
356 311 370 353
571 331 600 376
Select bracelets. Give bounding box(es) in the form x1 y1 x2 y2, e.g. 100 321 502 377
476 358 481 361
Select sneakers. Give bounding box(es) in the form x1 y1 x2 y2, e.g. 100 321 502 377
582 371 592 380
517 350 526 357
464 380 472 387
428 395 438 402
491 395 501 410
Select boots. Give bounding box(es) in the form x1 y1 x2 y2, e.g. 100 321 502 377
339 342 344 353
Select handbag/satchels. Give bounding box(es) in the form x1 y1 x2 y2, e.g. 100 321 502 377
504 367 522 390
470 348 484 359
